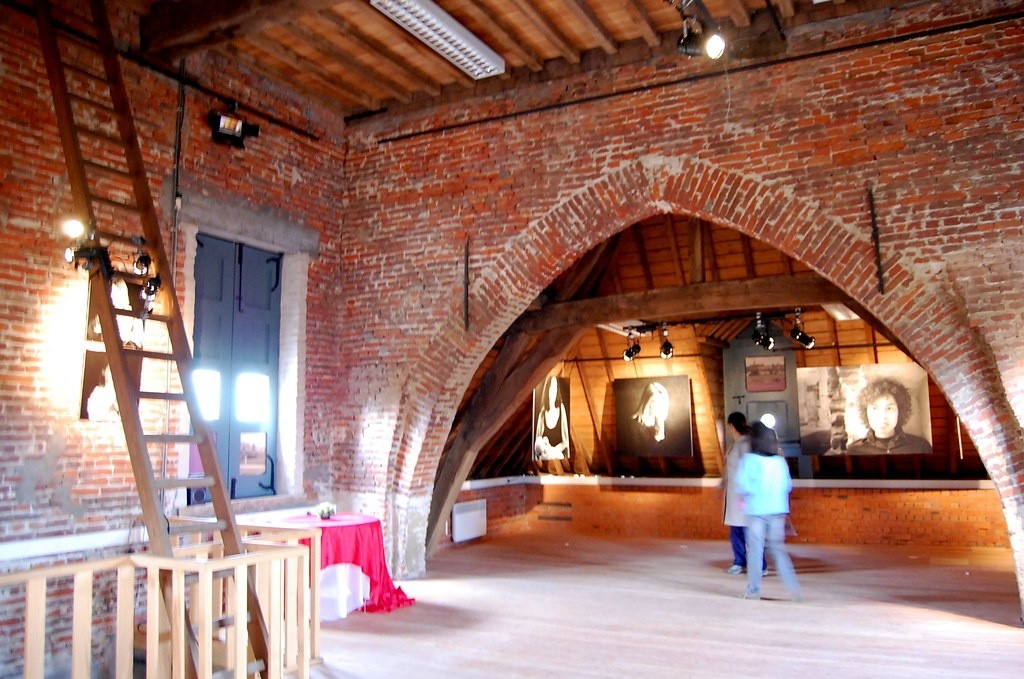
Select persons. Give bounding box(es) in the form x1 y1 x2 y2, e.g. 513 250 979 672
534 376 570 461
723 411 803 601
845 377 932 455
628 382 680 477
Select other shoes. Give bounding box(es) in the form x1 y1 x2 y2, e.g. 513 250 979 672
739 590 761 601
763 567 768 576
728 564 743 575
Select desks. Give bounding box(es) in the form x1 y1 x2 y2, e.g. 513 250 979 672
253 512 416 614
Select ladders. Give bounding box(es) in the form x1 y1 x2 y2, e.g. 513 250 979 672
30 0 270 679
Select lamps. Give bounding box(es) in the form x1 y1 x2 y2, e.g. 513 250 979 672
133 254 150 276
675 0 725 60
751 312 775 350
789 308 815 349
659 323 674 359
140 278 158 302
622 330 642 362
64 244 92 263
370 0 504 80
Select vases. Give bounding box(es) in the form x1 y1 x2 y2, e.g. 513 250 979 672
321 512 331 519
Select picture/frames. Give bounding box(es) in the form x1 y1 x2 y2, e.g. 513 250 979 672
744 355 786 392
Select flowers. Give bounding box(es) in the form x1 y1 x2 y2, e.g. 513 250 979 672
315 502 337 516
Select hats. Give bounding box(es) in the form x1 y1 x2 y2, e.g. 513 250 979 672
727 411 746 424
758 428 777 454
